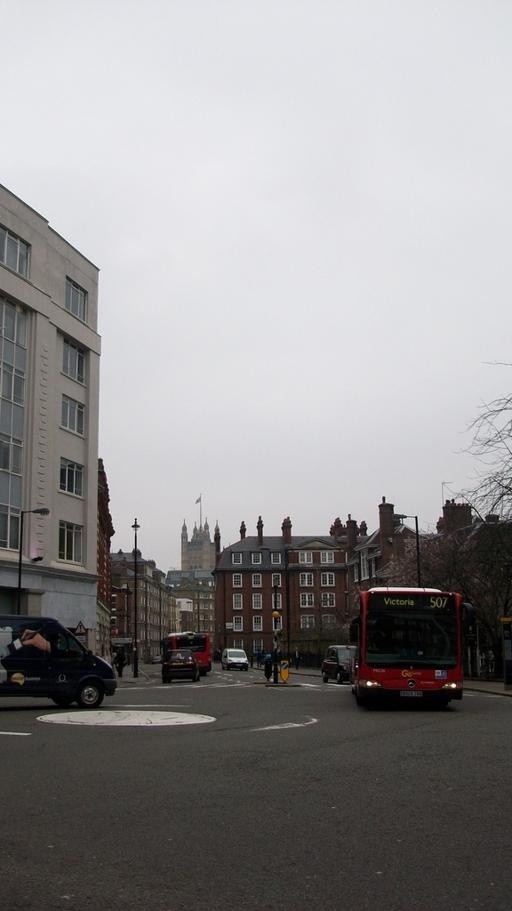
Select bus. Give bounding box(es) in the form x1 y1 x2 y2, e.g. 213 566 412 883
160 631 214 678
349 587 467 713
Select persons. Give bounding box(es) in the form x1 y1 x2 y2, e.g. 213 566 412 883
21 628 56 657
212 646 302 671
112 648 126 679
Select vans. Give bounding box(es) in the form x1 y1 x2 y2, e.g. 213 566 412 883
1 614 119 710
221 648 250 672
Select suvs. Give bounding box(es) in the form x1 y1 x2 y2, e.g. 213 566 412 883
321 644 359 686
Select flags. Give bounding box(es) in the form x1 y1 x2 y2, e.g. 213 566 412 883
194 494 201 505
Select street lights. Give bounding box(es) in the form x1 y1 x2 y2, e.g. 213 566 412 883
390 512 424 587
270 584 283 684
158 570 164 655
16 508 53 616
196 579 203 633
130 517 144 678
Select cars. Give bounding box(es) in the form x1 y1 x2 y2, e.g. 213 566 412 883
162 648 202 686
152 656 163 666
